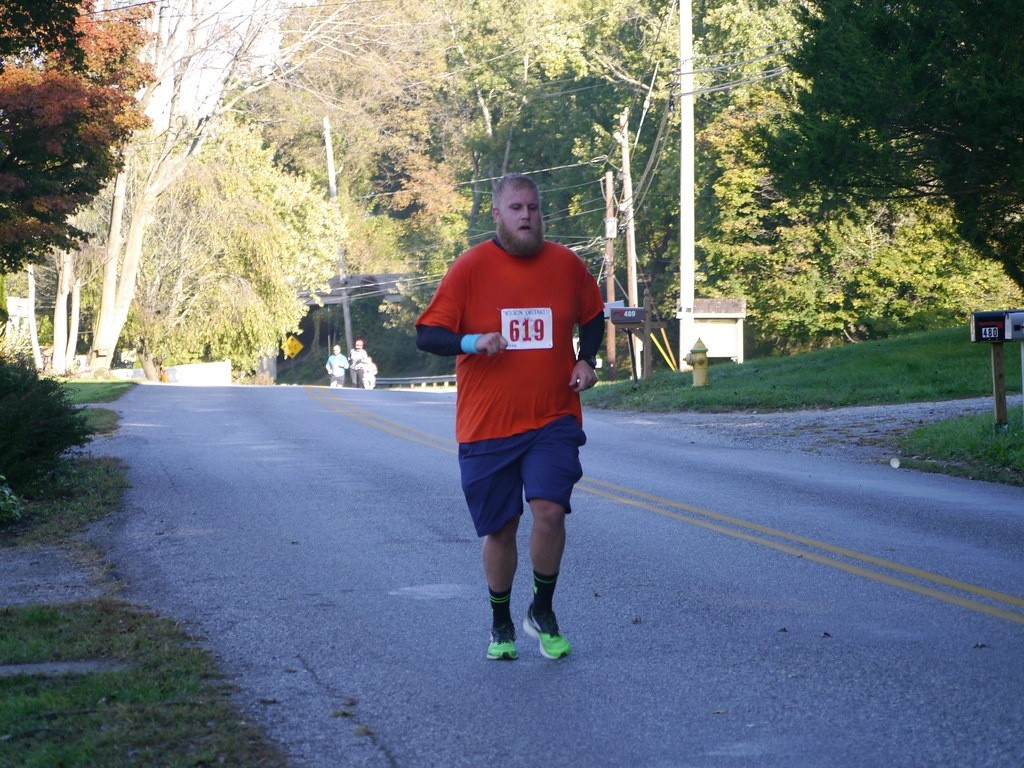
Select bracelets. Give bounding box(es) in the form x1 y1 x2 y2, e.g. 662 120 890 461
461 333 482 355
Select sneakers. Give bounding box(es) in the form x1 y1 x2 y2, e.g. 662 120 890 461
522 604 570 659
487 621 518 660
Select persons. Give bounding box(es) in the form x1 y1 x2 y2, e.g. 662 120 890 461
415 174 605 660
325 340 378 390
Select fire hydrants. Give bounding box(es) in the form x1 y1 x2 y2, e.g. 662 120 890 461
683 337 710 388
